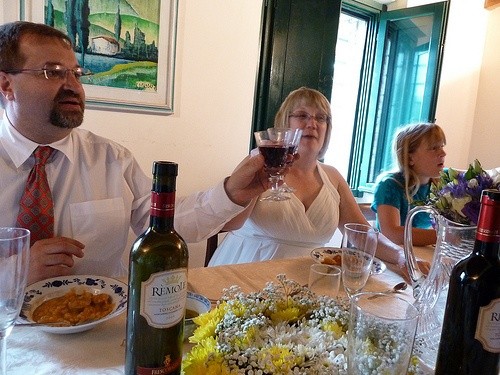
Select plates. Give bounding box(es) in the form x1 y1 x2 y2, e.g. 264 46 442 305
310 247 387 275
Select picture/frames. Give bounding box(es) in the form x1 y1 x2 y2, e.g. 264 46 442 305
17 0 179 115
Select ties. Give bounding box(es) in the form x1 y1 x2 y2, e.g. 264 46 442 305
14 146 55 254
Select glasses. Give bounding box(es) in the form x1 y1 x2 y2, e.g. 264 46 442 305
3 64 92 81
289 111 328 123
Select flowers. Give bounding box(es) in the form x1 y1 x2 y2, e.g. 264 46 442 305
182 273 426 375
429 159 496 225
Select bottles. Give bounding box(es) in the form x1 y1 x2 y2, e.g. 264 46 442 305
124 160 188 375
434 188 500 375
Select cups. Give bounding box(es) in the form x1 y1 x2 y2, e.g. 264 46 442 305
341 222 379 299
308 264 341 297
347 292 420 375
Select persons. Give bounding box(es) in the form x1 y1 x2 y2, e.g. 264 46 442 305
207 87 431 286
370 122 462 247
0 20 293 292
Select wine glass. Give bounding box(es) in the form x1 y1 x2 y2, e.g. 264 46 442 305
253 127 303 201
0 227 30 375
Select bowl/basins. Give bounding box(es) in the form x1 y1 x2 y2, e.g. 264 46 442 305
183 291 211 338
15 275 128 334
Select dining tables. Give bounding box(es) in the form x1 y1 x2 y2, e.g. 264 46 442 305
6 241 438 375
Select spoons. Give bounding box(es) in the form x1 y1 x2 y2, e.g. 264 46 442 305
368 282 408 300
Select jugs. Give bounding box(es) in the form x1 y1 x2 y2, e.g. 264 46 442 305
404 205 477 371
